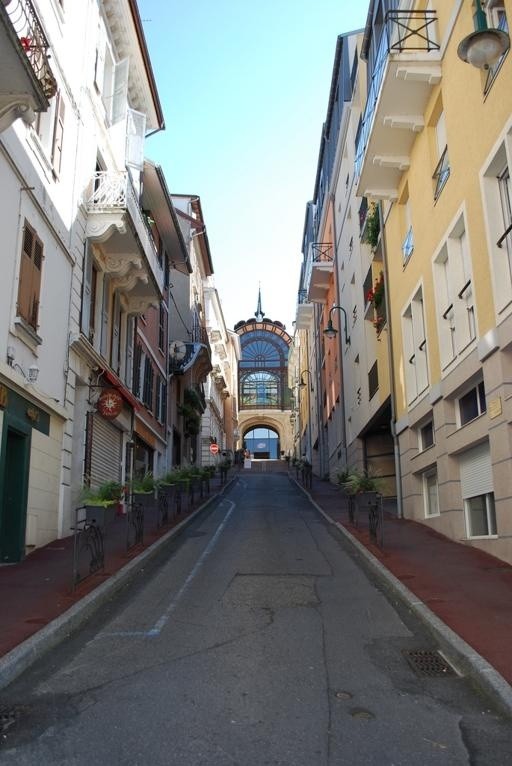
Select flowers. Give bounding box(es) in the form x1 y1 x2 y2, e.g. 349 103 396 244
368 269 385 304
373 315 385 333
366 201 380 244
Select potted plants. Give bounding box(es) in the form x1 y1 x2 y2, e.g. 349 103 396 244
340 466 383 508
84 465 216 567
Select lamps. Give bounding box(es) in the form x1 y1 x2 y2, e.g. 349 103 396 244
7 346 39 384
457 0 510 70
298 369 314 392
323 306 350 346
